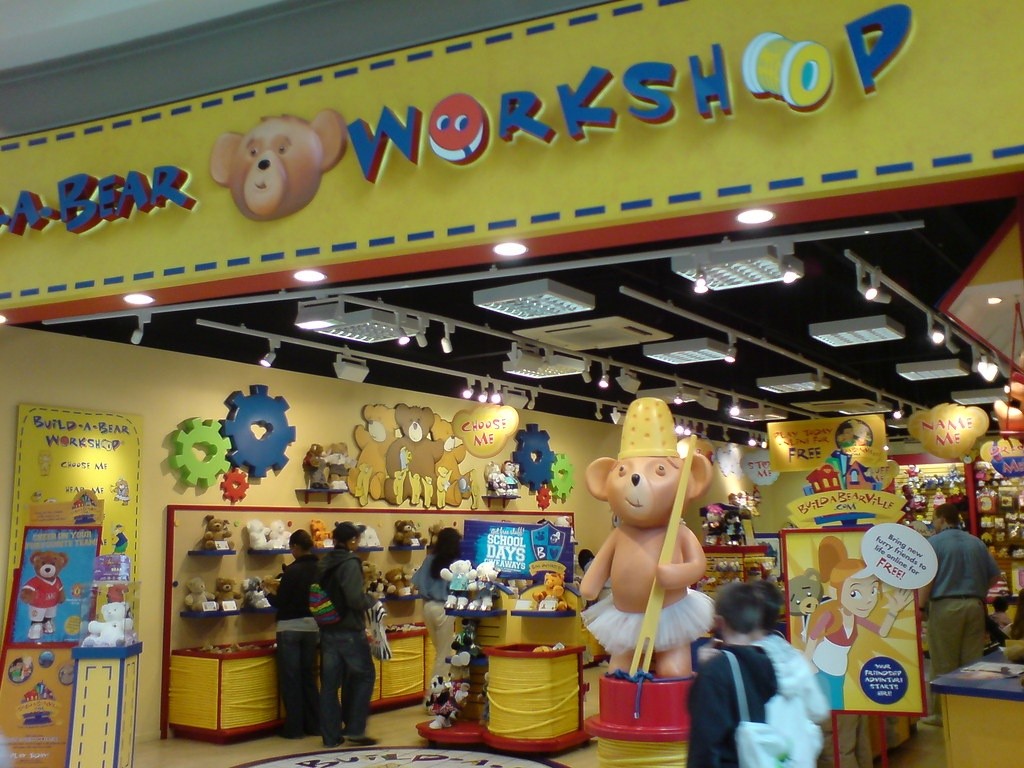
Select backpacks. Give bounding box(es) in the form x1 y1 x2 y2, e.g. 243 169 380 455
308 556 363 628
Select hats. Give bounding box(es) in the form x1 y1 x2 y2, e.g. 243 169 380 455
333 521 366 542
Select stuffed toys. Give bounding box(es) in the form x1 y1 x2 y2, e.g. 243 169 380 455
975 461 1024 560
309 518 333 549
706 561 742 585
484 461 518 496
213 577 242 611
20 552 68 639
361 561 420 599
80 601 133 648
901 464 965 530
533 572 567 612
429 519 444 544
302 442 349 489
440 560 501 611
425 618 482 728
185 576 219 611
203 514 234 550
702 506 745 546
393 520 427 546
241 575 280 609
200 644 262 654
246 518 291 550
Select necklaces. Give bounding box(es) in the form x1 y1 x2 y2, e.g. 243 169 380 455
941 526 960 531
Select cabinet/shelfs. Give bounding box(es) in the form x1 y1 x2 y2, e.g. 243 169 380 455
177 545 423 616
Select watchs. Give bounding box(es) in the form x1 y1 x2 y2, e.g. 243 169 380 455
918 606 926 612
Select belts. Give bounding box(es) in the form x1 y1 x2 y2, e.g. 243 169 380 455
930 595 977 601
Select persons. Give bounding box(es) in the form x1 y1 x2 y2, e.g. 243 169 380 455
917 502 1000 726
273 521 378 748
990 596 1012 628
685 579 830 768
1001 587 1024 641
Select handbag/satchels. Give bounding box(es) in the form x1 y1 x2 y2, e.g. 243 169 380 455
720 642 824 768
365 600 392 660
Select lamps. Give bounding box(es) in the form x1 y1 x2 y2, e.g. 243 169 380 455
129 246 1013 437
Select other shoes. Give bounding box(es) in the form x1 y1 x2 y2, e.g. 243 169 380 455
348 736 376 744
275 726 304 739
324 737 345 748
303 724 321 735
923 714 943 726
